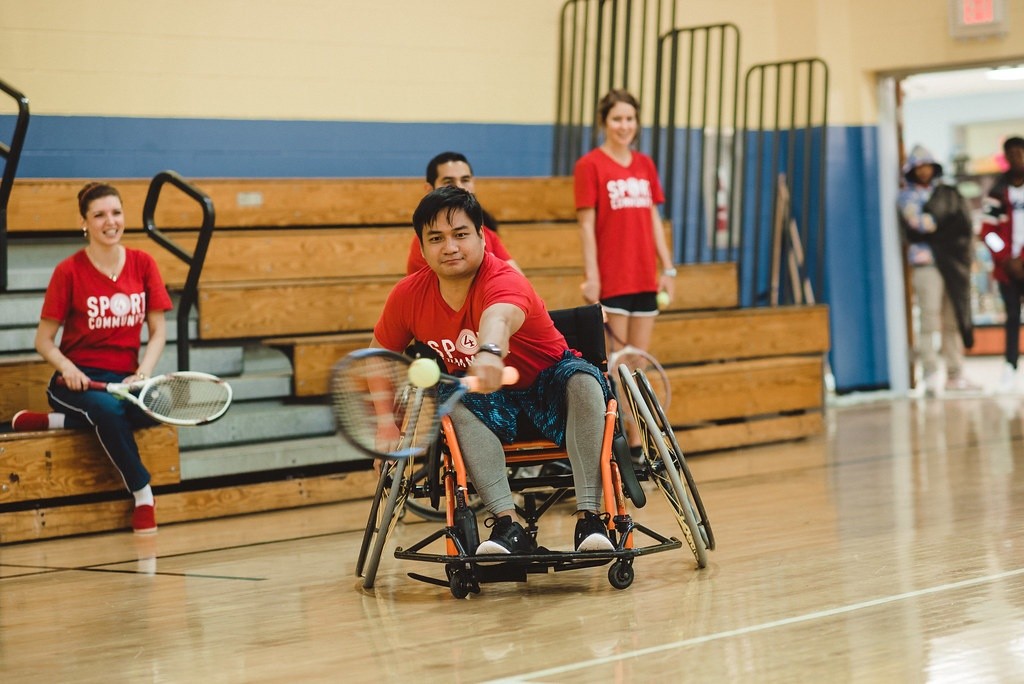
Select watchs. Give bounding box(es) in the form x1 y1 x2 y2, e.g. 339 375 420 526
476 343 503 359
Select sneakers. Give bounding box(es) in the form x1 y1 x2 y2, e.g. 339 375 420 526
475 510 538 565
571 509 615 551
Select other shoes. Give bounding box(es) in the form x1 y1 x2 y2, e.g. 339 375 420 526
12 410 49 432
133 499 157 533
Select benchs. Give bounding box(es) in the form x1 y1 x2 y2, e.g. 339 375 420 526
0 172 830 548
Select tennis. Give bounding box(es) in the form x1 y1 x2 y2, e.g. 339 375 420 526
656 292 672 311
406 356 439 388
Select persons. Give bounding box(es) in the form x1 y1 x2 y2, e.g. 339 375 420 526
362 183 617 566
977 136 1024 388
573 90 679 465
896 144 986 401
11 182 174 535
406 152 526 284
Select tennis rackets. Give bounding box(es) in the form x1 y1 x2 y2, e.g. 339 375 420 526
582 283 671 414
54 371 233 425
327 349 519 461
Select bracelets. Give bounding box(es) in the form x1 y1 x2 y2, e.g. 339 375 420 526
662 268 677 278
134 371 149 381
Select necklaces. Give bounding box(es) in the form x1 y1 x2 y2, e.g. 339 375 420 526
88 244 120 283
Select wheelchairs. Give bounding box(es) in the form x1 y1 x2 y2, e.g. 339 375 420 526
355 304 714 600
